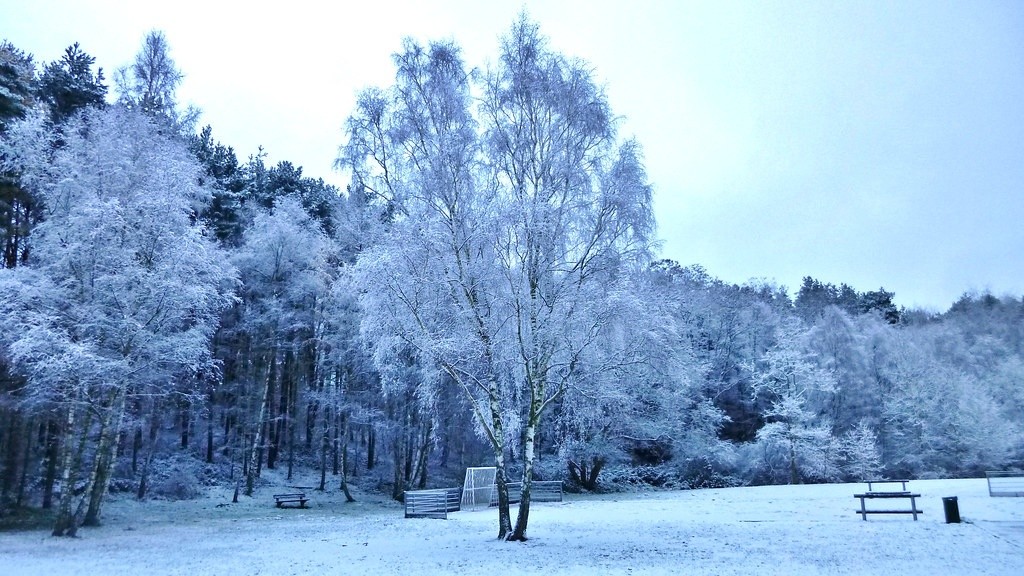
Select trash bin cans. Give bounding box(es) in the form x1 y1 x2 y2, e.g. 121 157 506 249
941 496 961 524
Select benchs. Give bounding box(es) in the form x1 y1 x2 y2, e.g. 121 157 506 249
274 493 310 509
854 479 923 521
